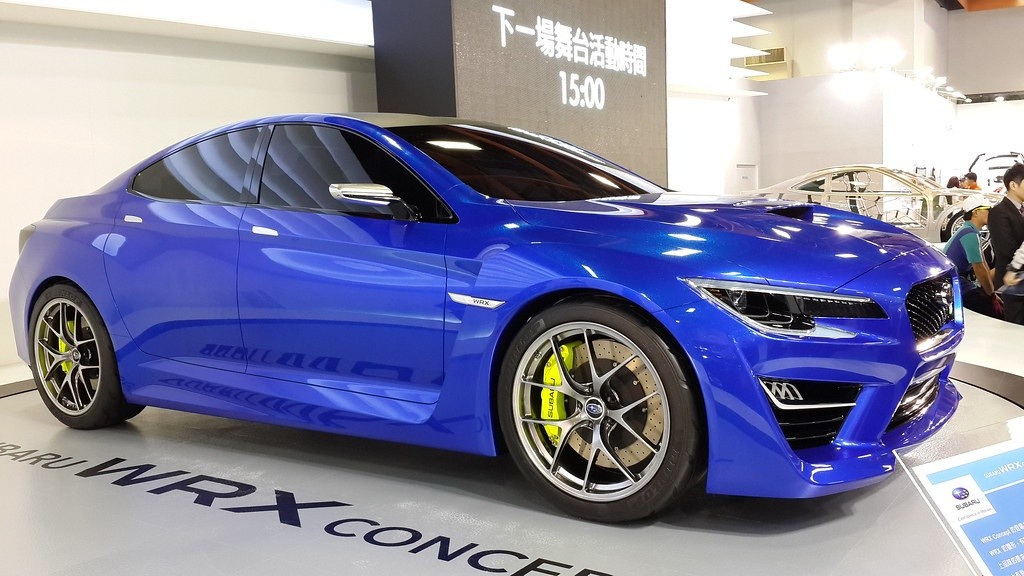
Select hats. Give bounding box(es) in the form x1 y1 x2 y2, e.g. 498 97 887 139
963 195 992 212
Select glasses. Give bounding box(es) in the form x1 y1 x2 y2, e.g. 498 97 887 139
972 207 991 212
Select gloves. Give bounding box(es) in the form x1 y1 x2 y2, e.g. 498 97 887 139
991 293 1004 314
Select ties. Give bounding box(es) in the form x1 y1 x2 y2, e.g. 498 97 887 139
1019 204 1024 216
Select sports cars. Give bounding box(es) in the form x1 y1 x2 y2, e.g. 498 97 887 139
741 164 1009 271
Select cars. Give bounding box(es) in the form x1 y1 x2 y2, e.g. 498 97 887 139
8 112 966 527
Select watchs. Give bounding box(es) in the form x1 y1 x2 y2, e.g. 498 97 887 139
1006 264 1020 272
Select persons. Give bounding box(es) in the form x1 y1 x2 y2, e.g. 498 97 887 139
946 172 980 205
988 164 1024 327
943 194 1004 320
